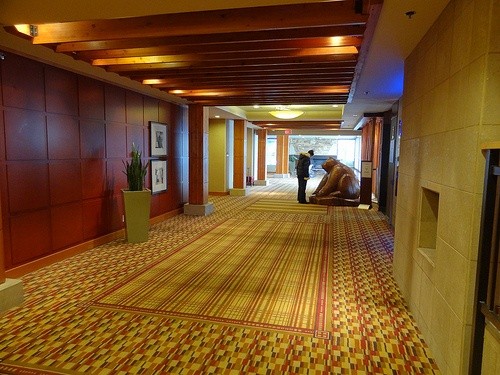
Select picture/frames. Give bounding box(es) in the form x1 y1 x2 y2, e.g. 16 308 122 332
150 121 169 194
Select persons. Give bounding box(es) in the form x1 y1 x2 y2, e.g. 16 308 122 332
296 150 314 204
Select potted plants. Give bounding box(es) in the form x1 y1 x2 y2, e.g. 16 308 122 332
121 144 152 244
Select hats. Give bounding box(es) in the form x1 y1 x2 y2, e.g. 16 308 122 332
308 150 314 156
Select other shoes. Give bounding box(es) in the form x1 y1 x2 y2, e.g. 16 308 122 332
299 201 310 204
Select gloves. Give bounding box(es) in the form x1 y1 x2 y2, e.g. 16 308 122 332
304 177 309 180
